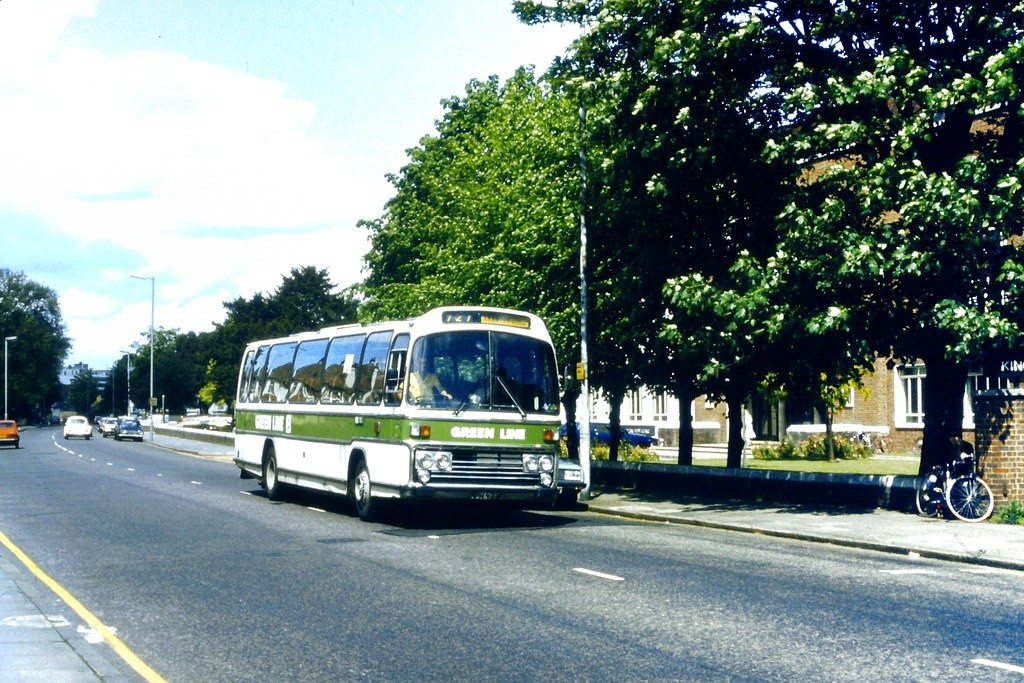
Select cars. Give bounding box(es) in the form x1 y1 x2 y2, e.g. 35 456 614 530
556 459 587 506
64 416 93 439
558 421 656 450
113 419 145 442
0 420 21 448
94 415 134 438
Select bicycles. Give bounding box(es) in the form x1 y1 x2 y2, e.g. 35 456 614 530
915 453 995 523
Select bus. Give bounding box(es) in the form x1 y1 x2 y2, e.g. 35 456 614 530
233 305 560 519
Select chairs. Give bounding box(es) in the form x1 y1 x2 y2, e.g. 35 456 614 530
243 370 543 414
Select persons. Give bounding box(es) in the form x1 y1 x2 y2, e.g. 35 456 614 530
948 433 979 519
396 357 453 408
